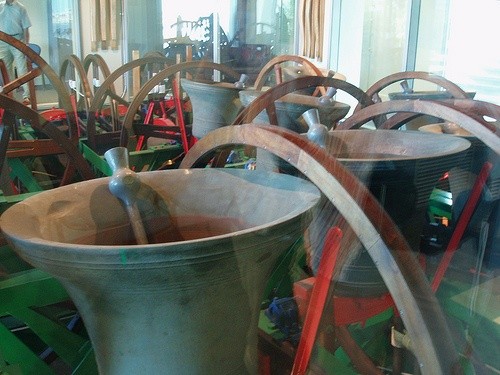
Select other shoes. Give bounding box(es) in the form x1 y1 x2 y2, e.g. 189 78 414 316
23 97 31 105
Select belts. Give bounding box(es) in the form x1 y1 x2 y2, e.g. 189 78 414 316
9 33 20 36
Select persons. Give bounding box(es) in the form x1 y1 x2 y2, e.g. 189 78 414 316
0 0 32 104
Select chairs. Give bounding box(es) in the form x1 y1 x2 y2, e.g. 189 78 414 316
28 43 46 88
